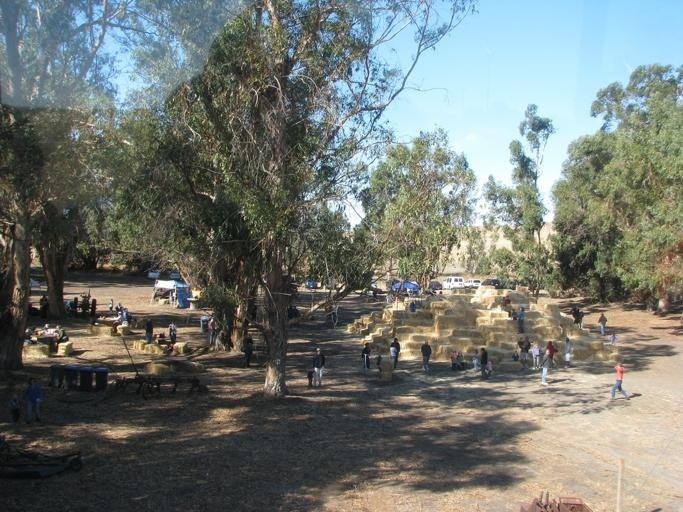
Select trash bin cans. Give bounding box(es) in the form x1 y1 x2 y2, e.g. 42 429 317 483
50 364 110 391
201 316 209 332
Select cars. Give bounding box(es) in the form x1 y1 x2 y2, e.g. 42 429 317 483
169 272 181 280
147 269 160 279
305 279 317 289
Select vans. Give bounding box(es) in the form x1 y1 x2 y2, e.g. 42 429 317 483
464 279 481 289
442 277 465 290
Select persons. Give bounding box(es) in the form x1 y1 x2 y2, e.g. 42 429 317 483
504 296 510 304
500 297 505 306
360 343 369 368
510 309 517 320
516 307 524 334
610 362 631 402
23 378 43 424
312 348 324 387
576 309 582 329
609 333 615 345
420 340 430 371
247 333 252 344
389 337 400 369
167 319 176 344
23 292 134 354
5 394 21 426
597 314 606 336
572 307 578 325
408 300 415 312
207 318 215 345
145 319 153 344
449 336 575 381
243 339 252 367
431 287 436 294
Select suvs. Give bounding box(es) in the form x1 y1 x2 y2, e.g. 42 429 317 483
481 279 500 289
